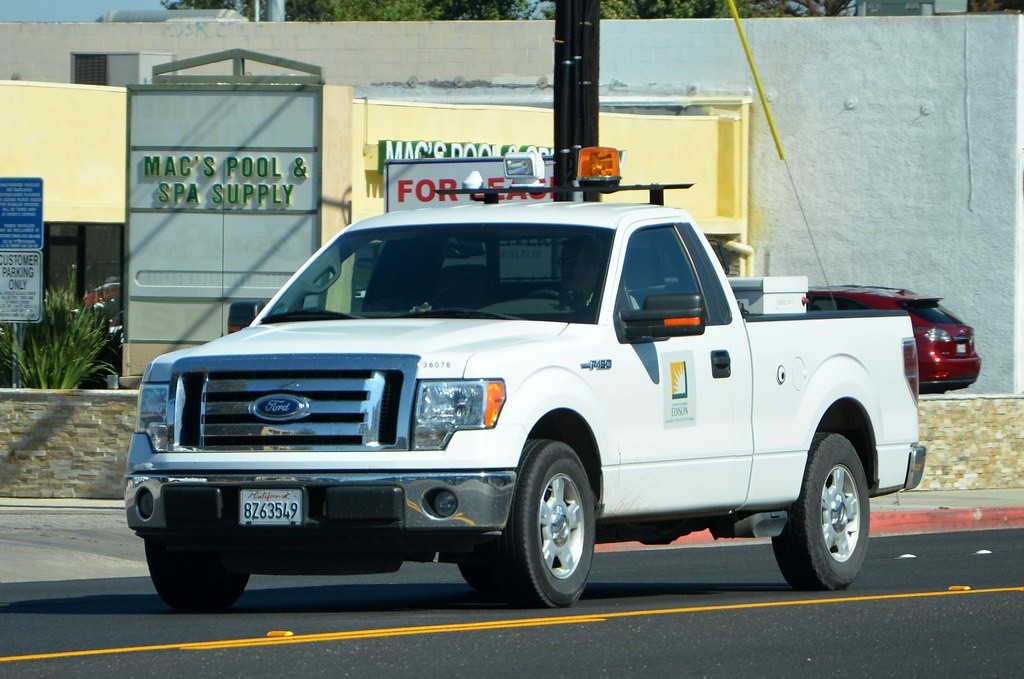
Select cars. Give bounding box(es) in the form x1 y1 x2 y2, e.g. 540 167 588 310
807 284 982 394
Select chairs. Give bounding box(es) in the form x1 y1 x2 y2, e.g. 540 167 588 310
435 264 497 312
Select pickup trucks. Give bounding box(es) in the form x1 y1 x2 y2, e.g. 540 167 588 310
123 144 927 611
